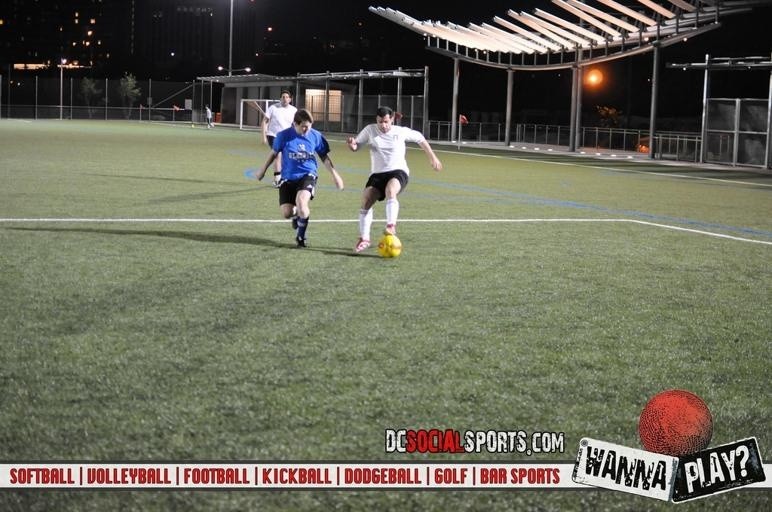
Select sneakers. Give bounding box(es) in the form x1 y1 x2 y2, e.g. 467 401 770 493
273 174 281 188
207 124 214 128
292 218 298 229
384 223 395 235
296 236 306 248
355 237 371 252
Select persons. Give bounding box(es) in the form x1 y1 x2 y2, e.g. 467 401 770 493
205 104 214 129
261 90 298 185
255 108 344 247
345 106 442 254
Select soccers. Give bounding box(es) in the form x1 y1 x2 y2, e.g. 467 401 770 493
377 234 402 258
